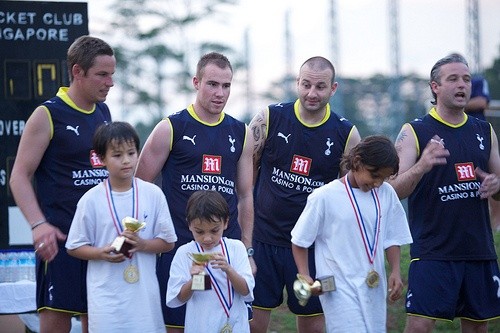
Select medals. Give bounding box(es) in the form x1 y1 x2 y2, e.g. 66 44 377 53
125 264 138 281
220 327 234 333
363 267 383 290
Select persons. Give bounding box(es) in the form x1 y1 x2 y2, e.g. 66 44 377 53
248 56 361 333
290 135 413 333
386 54 500 333
65 123 177 333
170 190 256 333
10 35 116 333
447 55 500 235
134 52 256 333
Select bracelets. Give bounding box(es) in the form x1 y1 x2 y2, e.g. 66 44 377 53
30 218 48 231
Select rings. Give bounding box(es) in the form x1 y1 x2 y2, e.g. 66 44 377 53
39 242 47 249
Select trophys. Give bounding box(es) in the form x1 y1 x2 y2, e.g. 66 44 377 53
112 217 146 258
187 251 216 291
291 273 338 305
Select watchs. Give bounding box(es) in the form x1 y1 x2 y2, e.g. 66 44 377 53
245 248 256 255
